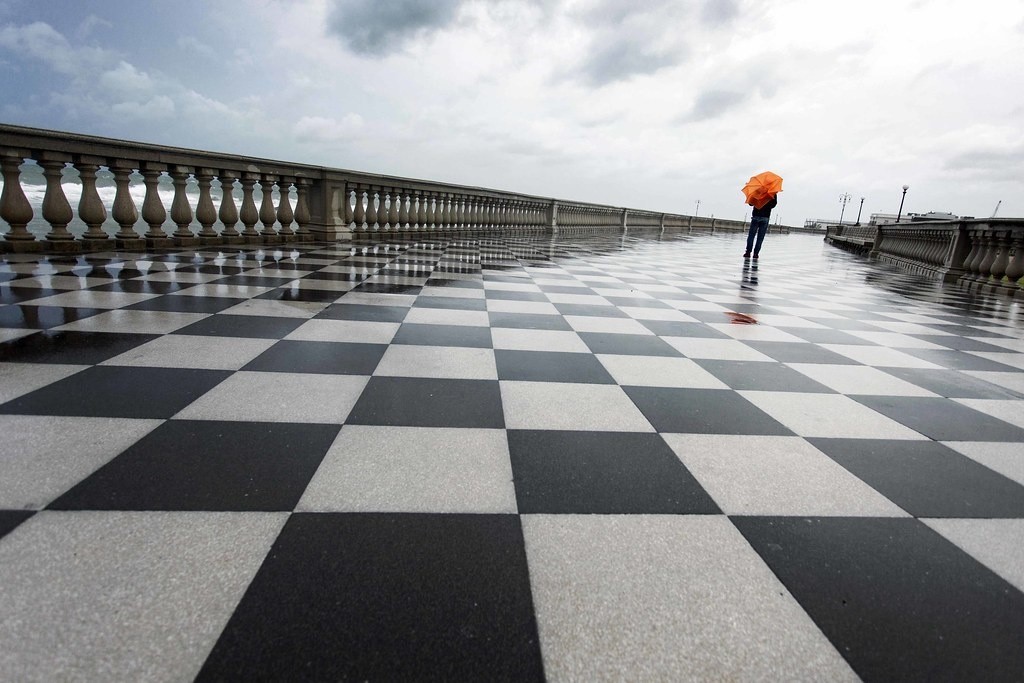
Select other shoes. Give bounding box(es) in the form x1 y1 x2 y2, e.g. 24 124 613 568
753 253 758 258
743 252 750 257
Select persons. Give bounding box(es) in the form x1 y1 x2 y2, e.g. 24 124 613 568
742 191 777 258
742 256 760 292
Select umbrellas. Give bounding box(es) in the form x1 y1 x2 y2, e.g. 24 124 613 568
741 171 783 210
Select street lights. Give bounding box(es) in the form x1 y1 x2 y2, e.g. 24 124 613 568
894 183 909 223
838 192 852 225
854 197 865 227
991 200 1001 217
696 200 701 216
